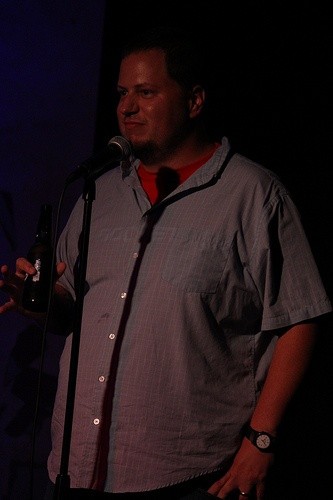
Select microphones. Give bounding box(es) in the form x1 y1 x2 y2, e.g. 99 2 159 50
65 135 132 184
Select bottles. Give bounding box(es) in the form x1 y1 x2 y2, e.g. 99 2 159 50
21 204 56 313
